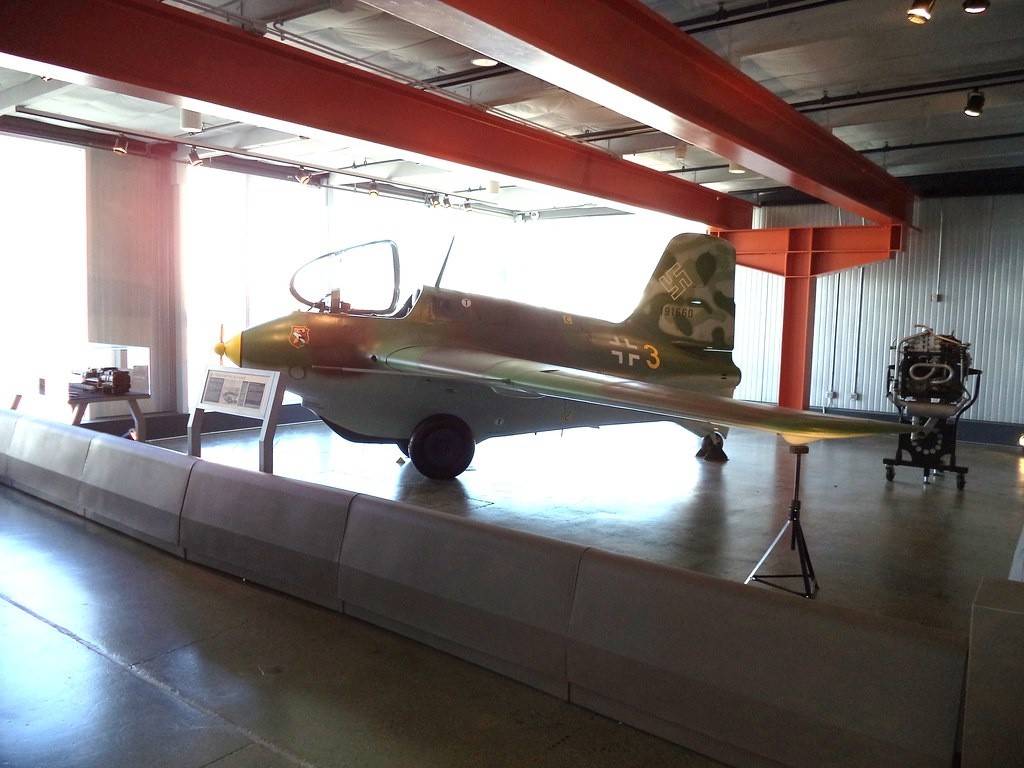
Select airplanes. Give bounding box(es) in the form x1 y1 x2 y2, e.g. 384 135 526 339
212 230 924 483
